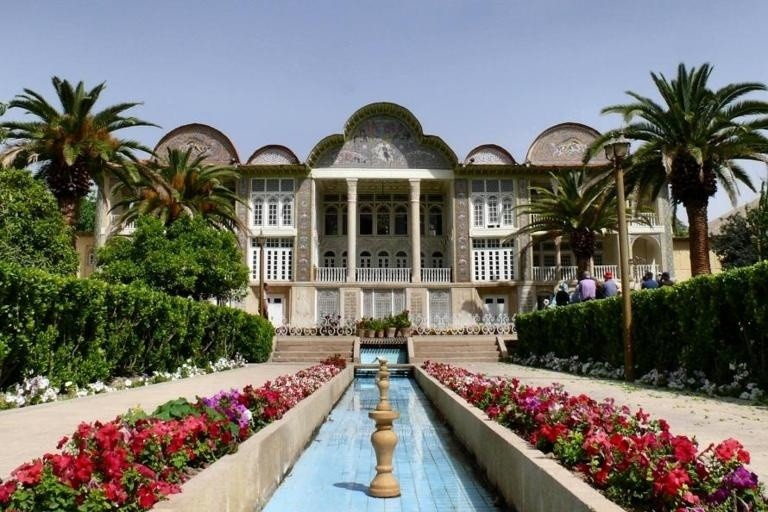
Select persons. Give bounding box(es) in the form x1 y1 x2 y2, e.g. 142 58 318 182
258 282 268 320
639 271 674 288
554 271 619 306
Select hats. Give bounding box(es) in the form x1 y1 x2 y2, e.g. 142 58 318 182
646 272 670 277
583 271 613 278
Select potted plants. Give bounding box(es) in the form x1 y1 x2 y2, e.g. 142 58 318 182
355 311 413 338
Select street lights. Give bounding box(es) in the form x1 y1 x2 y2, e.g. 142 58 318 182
604 125 635 382
256 228 267 316
